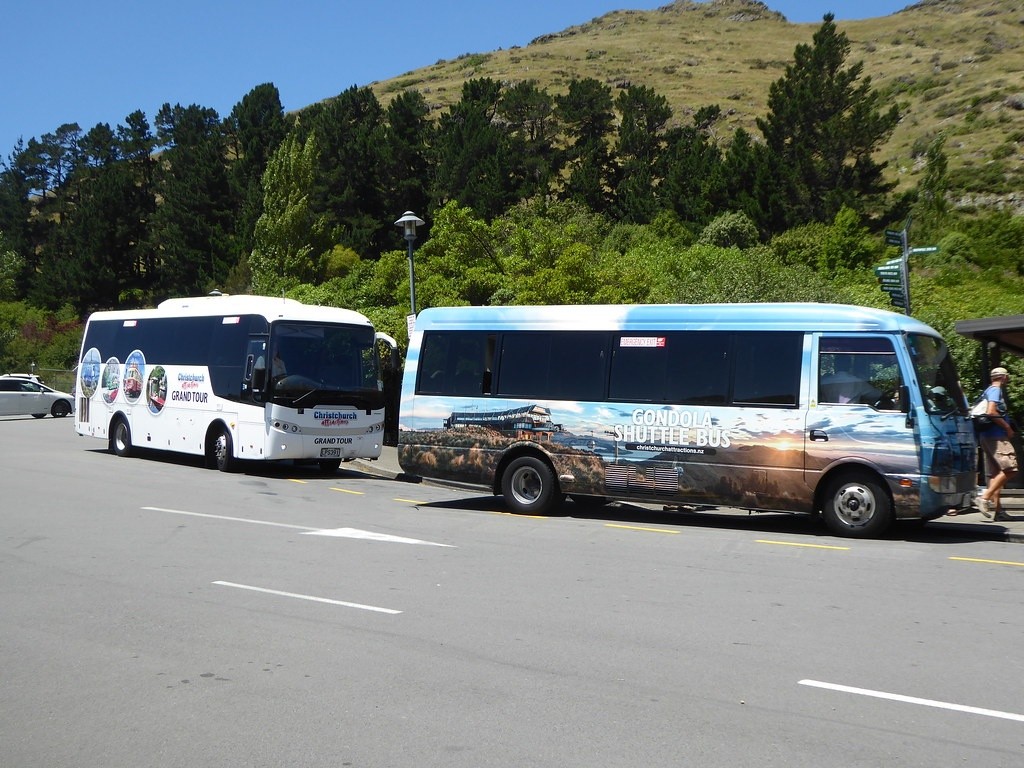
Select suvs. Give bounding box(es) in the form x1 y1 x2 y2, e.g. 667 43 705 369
3 373 45 392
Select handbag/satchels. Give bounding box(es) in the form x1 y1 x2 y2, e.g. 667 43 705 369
968 386 996 428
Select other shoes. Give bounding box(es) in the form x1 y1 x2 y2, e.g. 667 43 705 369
947 508 957 516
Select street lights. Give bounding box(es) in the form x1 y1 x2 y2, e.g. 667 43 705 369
393 209 424 315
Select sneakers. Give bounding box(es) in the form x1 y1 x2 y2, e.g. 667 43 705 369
994 512 1015 521
973 497 991 518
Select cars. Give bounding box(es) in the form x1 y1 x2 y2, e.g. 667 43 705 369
0 377 75 418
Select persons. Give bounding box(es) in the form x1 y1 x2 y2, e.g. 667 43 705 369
948 508 957 516
820 355 884 409
895 391 899 403
974 368 1018 520
255 344 287 383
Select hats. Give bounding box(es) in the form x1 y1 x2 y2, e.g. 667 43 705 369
991 367 1010 376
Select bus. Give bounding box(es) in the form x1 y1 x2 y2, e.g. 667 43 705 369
397 303 1014 539
107 373 119 390
125 367 142 397
74 295 402 473
